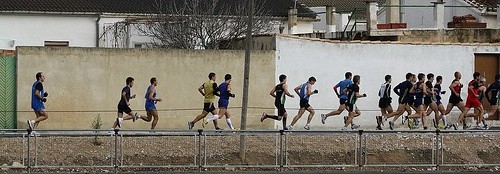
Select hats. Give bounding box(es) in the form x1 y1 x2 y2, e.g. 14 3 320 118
480 77 486 81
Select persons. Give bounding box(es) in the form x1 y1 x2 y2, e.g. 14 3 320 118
287 76 318 134
433 72 472 130
27 72 48 137
261 74 294 134
202 74 240 133
408 73 446 129
452 72 485 129
478 74 500 126
321 72 362 129
341 75 366 131
382 73 419 129
376 75 393 130
416 76 453 130
402 73 431 130
464 77 494 130
389 74 418 130
133 77 163 133
188 72 223 133
109 77 136 133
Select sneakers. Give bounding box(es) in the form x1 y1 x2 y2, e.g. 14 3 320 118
402 115 406 125
28 120 34 130
389 120 394 130
202 117 208 128
376 126 382 130
477 122 489 129
453 124 458 130
233 128 240 133
382 116 385 126
304 124 311 130
482 117 486 125
415 117 419 126
463 126 471 129
261 112 267 122
321 114 327 124
351 124 360 129
117 118 123 128
188 122 194 129
108 128 119 134
433 119 437 128
30 131 40 136
376 116 382 126
344 116 348 124
341 128 348 131
444 124 452 129
288 124 293 134
133 112 139 122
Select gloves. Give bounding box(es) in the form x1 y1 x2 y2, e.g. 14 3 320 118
231 94 235 98
441 91 446 94
363 94 366 97
314 90 318 93
44 92 48 97
41 97 46 102
428 92 433 97
